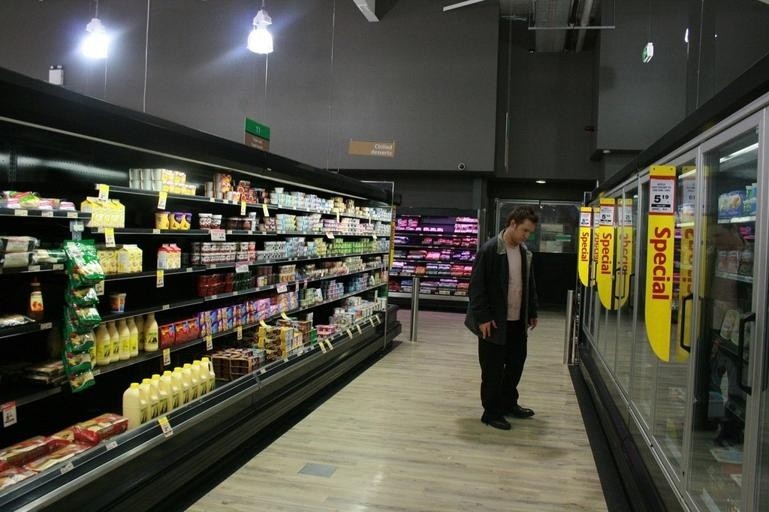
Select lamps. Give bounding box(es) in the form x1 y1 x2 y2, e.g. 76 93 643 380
249 1 274 54
86 1 107 53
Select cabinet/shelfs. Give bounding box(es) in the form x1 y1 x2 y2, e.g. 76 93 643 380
389 208 481 303
1 68 401 512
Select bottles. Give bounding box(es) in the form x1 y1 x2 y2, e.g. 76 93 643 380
76 311 158 365
28 277 45 323
196 271 252 297
187 241 287 263
254 264 297 285
228 212 258 230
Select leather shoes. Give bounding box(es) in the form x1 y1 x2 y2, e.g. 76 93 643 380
505 404 534 417
481 418 511 429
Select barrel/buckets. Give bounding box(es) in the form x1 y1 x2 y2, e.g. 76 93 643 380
121 356 217 429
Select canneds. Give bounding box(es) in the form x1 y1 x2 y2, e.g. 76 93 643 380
254 264 279 288
233 271 256 291
181 253 189 266
188 242 256 266
279 264 296 283
197 272 233 298
228 216 252 231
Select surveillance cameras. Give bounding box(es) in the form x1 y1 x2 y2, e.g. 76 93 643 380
458 162 466 171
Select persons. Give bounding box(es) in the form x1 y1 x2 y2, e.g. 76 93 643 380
692 209 754 448
464 205 541 430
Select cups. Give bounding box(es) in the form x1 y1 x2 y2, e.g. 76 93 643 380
110 292 127 313
152 211 223 231
